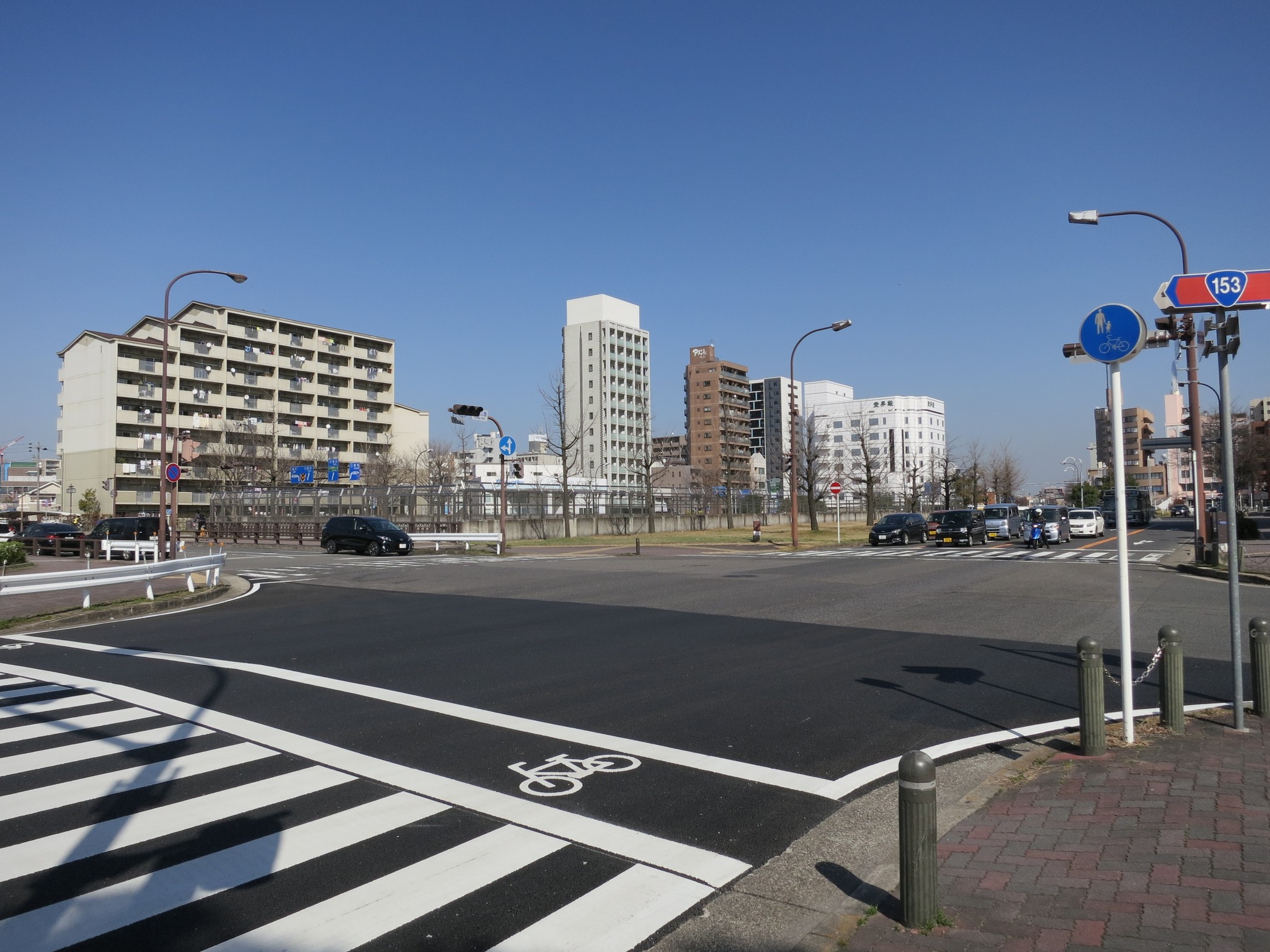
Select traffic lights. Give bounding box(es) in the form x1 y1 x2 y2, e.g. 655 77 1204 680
1180 418 1193 437
514 462 525 479
1153 313 1175 334
454 403 483 417
219 464 234 470
102 479 109 491
182 439 201 463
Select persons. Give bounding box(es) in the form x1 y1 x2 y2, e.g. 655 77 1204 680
1025 508 1050 549
72 516 82 528
193 509 208 537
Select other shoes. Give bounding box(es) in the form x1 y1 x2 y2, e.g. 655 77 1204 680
1027 546 1030 548
1047 546 1050 549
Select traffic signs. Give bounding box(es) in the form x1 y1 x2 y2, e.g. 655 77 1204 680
290 465 315 484
328 459 339 482
348 463 360 482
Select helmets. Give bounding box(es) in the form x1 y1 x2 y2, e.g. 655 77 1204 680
1035 508 1042 515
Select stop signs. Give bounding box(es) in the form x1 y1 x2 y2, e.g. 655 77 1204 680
830 482 842 494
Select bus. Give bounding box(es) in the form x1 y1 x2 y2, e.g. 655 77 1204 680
1103 486 1151 528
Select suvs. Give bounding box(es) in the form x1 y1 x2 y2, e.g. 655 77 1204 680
83 516 170 561
320 515 415 557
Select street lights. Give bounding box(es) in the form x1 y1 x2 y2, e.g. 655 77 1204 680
414 449 433 521
1074 459 1084 510
1087 448 1112 490
789 320 853 548
154 269 248 563
67 484 77 524
1067 210 1208 567
1061 457 1080 485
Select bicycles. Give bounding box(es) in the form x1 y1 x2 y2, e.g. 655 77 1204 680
194 522 208 537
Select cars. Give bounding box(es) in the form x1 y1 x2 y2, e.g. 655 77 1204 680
868 513 931 546
0 521 86 557
926 490 1104 552
1169 504 1195 517
